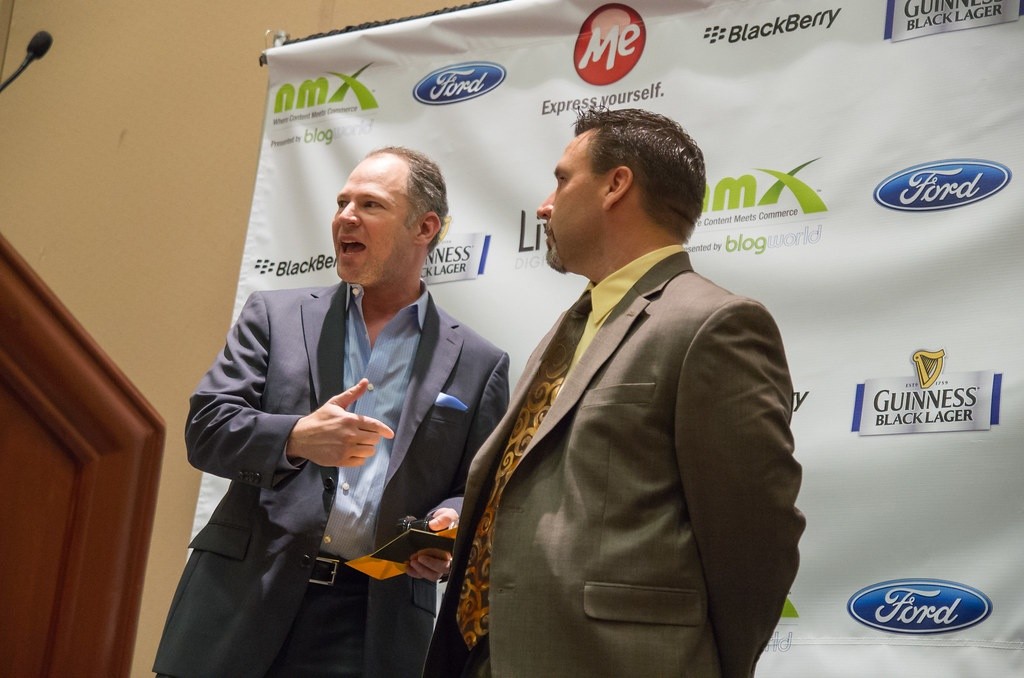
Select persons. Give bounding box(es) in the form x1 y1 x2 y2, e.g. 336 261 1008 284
419 105 808 678
152 143 510 678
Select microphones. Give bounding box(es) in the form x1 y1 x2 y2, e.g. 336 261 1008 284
0 30 54 92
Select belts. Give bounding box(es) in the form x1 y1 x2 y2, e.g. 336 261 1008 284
307 555 369 594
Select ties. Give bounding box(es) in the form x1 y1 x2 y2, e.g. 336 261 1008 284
455 289 592 650
